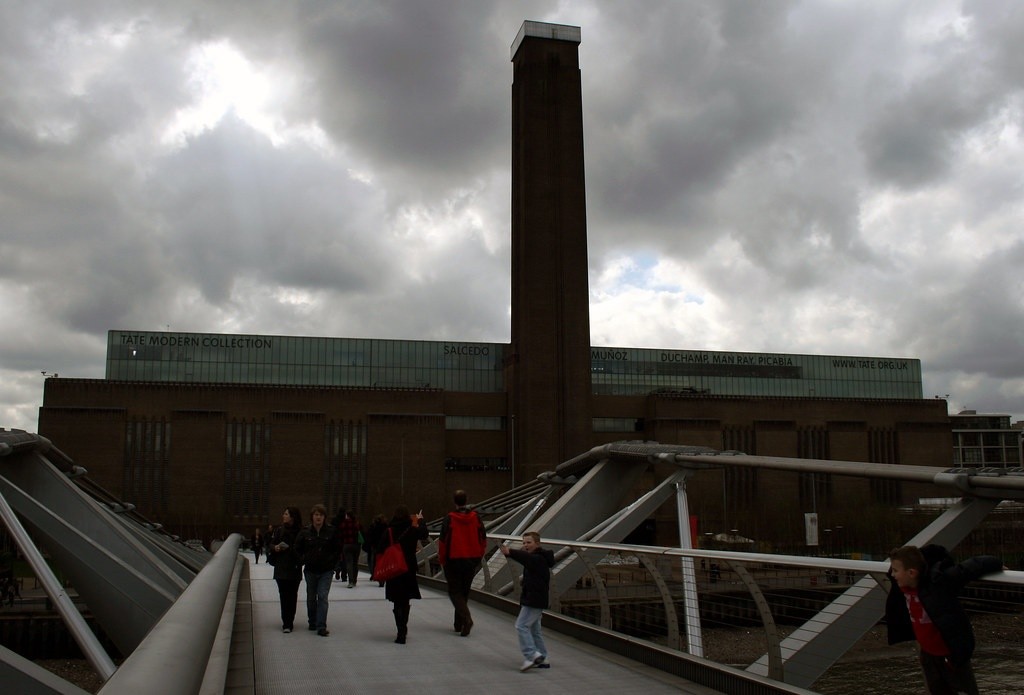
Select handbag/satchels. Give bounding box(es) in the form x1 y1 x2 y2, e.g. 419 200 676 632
357 521 365 544
269 529 280 566
373 528 408 581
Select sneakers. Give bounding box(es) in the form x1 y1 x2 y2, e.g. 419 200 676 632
520 651 546 671
530 657 550 668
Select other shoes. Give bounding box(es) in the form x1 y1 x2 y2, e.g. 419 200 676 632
337 576 340 580
455 624 461 632
370 576 375 582
309 622 317 630
283 628 290 633
395 626 407 644
347 583 356 588
318 627 329 635
342 579 347 582
378 584 384 587
461 618 473 637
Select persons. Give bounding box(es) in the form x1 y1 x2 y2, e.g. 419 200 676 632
884 544 1010 695
700 556 722 584
502 531 555 670
330 509 392 587
0 578 23 608
370 504 429 643
251 524 274 563
266 496 339 636
576 576 607 592
823 567 856 585
438 489 488 637
